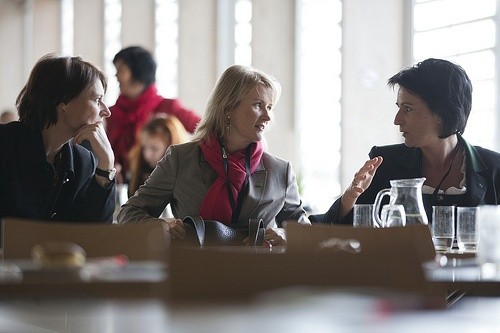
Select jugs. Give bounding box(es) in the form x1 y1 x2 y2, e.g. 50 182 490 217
372 177 428 228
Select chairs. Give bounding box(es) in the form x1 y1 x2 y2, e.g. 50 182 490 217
2 218 173 298
286 221 446 310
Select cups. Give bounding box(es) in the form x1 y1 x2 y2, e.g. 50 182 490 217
352 204 376 229
430 205 456 252
474 204 500 260
381 204 406 229
456 206 479 252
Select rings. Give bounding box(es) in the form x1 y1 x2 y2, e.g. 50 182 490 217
271 239 275 243
175 219 181 223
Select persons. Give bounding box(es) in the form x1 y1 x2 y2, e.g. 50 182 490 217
102 46 200 182
323 57 500 227
117 65 313 248
0 51 117 222
129 113 191 219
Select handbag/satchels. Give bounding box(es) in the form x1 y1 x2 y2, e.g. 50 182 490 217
180 216 264 246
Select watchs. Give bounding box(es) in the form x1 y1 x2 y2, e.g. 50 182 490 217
95 167 117 180
